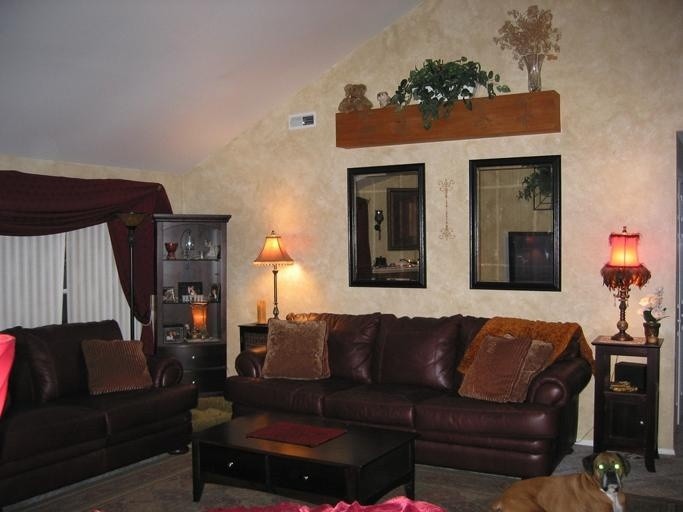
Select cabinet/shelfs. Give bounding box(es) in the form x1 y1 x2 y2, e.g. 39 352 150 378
150 213 231 395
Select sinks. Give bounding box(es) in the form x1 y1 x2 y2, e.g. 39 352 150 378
382 266 409 269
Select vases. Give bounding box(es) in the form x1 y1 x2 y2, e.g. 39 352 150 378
520 54 545 92
642 321 661 344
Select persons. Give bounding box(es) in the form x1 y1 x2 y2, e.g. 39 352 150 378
172 330 179 339
166 330 172 339
209 286 219 300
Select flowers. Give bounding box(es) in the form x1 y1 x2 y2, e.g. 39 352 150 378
637 287 669 321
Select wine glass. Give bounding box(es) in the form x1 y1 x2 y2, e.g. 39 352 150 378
165 242 177 259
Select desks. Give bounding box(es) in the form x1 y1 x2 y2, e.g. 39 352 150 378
235 322 270 352
582 333 665 473
371 264 419 282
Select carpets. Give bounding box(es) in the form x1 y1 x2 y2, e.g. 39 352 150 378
1 440 683 510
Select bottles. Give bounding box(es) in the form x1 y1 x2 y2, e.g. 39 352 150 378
256 299 266 323
374 255 418 270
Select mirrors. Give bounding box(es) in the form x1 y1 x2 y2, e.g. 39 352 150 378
466 153 564 294
345 162 427 291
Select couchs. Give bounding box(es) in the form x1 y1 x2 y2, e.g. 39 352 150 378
222 309 593 479
0 319 202 506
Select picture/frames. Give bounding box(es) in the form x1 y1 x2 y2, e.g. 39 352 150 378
385 186 419 250
162 285 178 304
161 324 184 344
508 231 553 284
176 281 203 303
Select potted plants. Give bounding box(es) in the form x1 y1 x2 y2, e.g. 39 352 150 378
387 54 513 135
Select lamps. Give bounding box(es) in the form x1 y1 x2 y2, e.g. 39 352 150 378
251 229 295 321
112 208 150 340
188 303 208 339
373 208 384 239
600 224 651 341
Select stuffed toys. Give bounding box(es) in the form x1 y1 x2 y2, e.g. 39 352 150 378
337 84 373 113
376 92 391 110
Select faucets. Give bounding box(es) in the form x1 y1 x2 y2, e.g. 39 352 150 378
399 257 417 265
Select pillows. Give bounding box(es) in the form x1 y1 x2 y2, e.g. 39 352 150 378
0 334 15 415
327 312 381 385
498 334 554 405
261 317 326 380
374 312 464 392
456 334 533 402
80 336 153 396
16 331 60 409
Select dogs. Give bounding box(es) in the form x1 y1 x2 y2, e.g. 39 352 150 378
490 451 631 512
